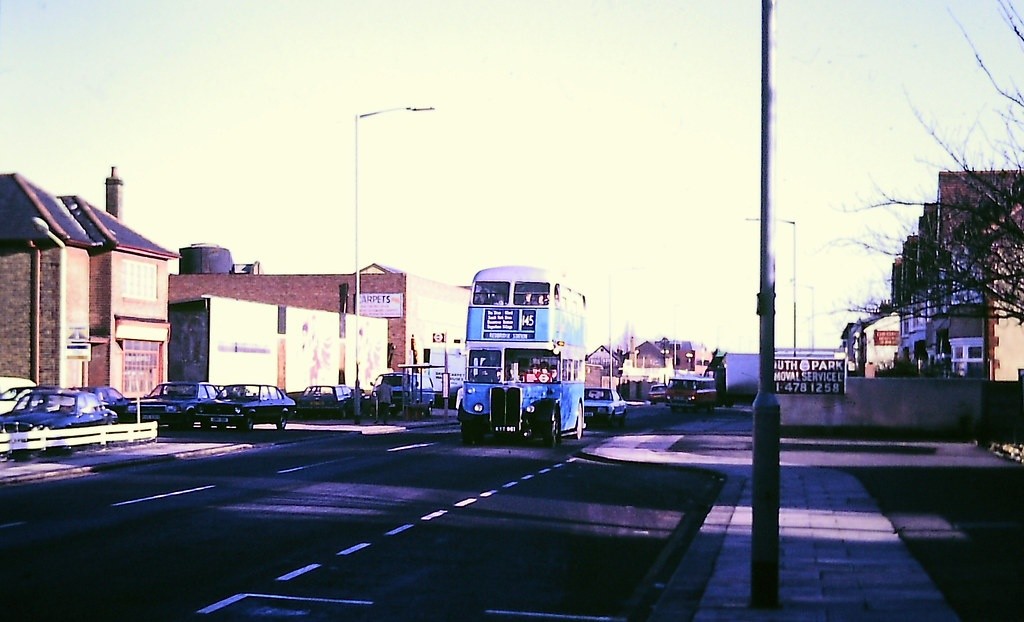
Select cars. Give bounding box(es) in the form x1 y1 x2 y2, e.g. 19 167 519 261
584 387 628 427
648 384 667 405
67 387 130 423
127 382 221 430
296 386 365 420
0 377 120 463
195 384 295 433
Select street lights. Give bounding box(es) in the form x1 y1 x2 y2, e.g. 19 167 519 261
351 106 435 424
748 218 798 358
32 215 66 386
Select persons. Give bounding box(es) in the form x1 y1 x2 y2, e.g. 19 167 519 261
477 289 555 308
478 358 575 387
374 378 392 425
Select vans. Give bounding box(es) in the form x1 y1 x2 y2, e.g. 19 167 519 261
369 373 436 416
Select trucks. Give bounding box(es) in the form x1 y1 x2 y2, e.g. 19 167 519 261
700 353 763 407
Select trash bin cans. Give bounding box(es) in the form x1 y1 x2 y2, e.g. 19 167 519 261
402 401 431 423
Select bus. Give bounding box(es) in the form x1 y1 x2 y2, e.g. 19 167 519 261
456 266 586 448
665 377 717 413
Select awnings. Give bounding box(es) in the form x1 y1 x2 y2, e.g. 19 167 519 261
1 173 181 260
